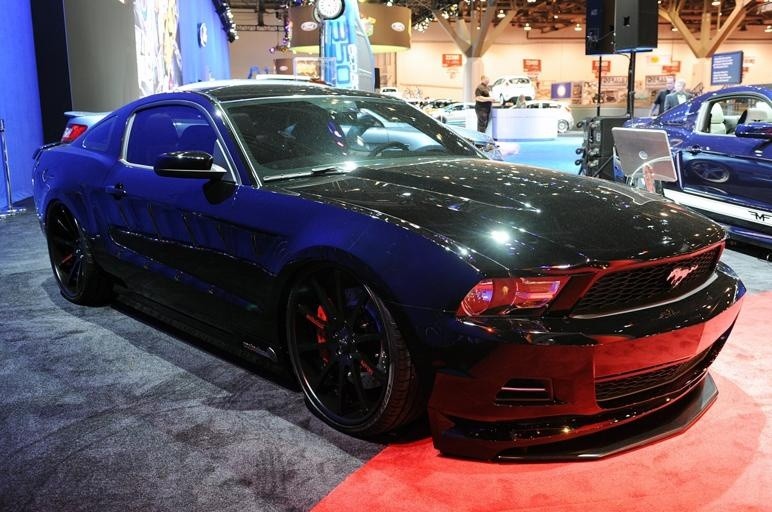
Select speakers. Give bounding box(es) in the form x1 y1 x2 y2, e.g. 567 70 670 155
586 0 658 54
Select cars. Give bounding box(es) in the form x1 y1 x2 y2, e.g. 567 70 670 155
509 100 575 133
613 83 772 254
57 74 504 162
489 75 535 105
381 86 476 124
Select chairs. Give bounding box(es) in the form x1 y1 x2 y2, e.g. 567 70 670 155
708 103 726 136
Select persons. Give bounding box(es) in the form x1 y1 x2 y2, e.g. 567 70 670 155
515 95 527 109
472 73 497 134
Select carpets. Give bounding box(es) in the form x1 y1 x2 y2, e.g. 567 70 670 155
307 286 772 512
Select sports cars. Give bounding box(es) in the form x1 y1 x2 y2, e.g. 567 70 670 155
677 139 772 187
31 80 745 465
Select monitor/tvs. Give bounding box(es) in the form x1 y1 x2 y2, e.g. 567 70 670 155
711 51 743 84
